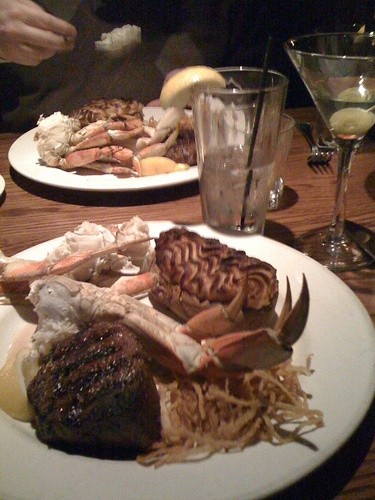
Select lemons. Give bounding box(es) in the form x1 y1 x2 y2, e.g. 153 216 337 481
160 64 226 109
0 348 39 421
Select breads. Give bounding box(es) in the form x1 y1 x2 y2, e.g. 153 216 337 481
145 227 279 335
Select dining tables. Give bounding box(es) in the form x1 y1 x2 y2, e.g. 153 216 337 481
1 106 375 500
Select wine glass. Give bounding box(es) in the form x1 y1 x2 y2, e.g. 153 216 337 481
283 33 375 271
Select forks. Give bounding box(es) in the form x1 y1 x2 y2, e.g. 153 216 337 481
296 122 334 175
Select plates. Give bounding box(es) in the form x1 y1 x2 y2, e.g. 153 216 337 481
0 175 5 194
8 107 199 191
0 220 375 500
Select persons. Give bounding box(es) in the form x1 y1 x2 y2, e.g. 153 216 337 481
0 0 77 68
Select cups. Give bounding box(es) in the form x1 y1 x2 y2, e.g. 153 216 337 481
190 66 289 237
217 109 299 211
